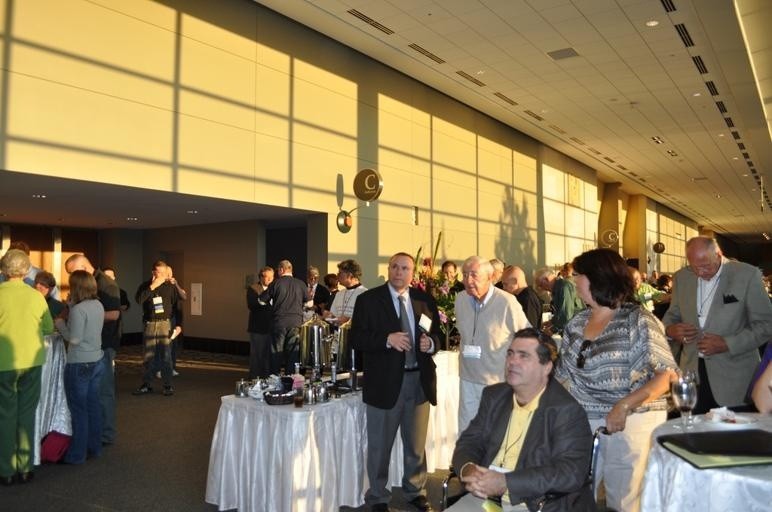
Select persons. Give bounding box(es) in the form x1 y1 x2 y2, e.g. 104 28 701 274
132 259 183 396
660 235 772 418
153 263 187 380
626 265 674 320
489 258 504 289
553 246 684 512
441 261 465 296
535 267 587 337
348 252 443 512
501 264 542 331
1 239 131 486
245 258 369 380
454 255 532 440
558 262 574 282
442 325 600 512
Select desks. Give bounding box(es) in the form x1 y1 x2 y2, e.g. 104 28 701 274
204 348 460 512
32 329 74 467
639 412 772 512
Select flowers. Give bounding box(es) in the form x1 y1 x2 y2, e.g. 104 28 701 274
410 230 461 351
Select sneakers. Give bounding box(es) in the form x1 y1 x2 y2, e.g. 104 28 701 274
133 368 180 396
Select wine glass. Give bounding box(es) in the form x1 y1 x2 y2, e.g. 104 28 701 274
670 367 698 428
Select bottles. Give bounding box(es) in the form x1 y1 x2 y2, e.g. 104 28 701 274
235 376 263 395
305 383 328 404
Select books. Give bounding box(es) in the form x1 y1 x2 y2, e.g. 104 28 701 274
655 428 772 470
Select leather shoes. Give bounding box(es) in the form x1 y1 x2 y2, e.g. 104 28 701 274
1 477 15 486
372 503 392 511
19 472 34 484
409 494 433 511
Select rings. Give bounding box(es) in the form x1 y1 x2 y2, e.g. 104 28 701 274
683 337 689 344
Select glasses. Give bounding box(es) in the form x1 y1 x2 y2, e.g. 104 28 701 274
685 255 719 272
460 271 481 282
576 339 592 368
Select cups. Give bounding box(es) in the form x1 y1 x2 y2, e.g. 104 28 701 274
293 387 304 407
459 462 489 479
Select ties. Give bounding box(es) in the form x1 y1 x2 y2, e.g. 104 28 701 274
398 296 418 372
